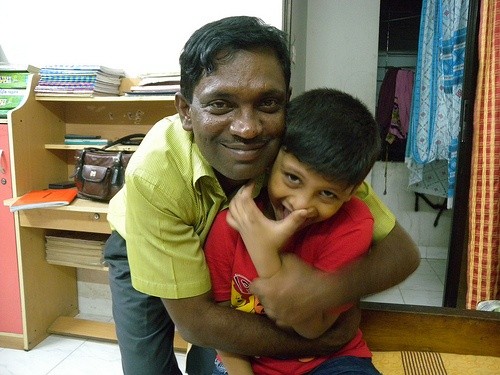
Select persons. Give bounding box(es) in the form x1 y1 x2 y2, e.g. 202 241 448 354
102 15 422 375
202 87 385 375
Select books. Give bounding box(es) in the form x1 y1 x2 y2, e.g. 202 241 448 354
40 232 114 276
0 60 182 150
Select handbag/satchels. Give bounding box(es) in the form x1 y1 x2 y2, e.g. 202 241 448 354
73 134 147 202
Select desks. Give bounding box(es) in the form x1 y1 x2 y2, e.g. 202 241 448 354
354 302 500 375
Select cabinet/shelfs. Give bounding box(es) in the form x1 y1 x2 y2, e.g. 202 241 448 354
0 63 237 355
